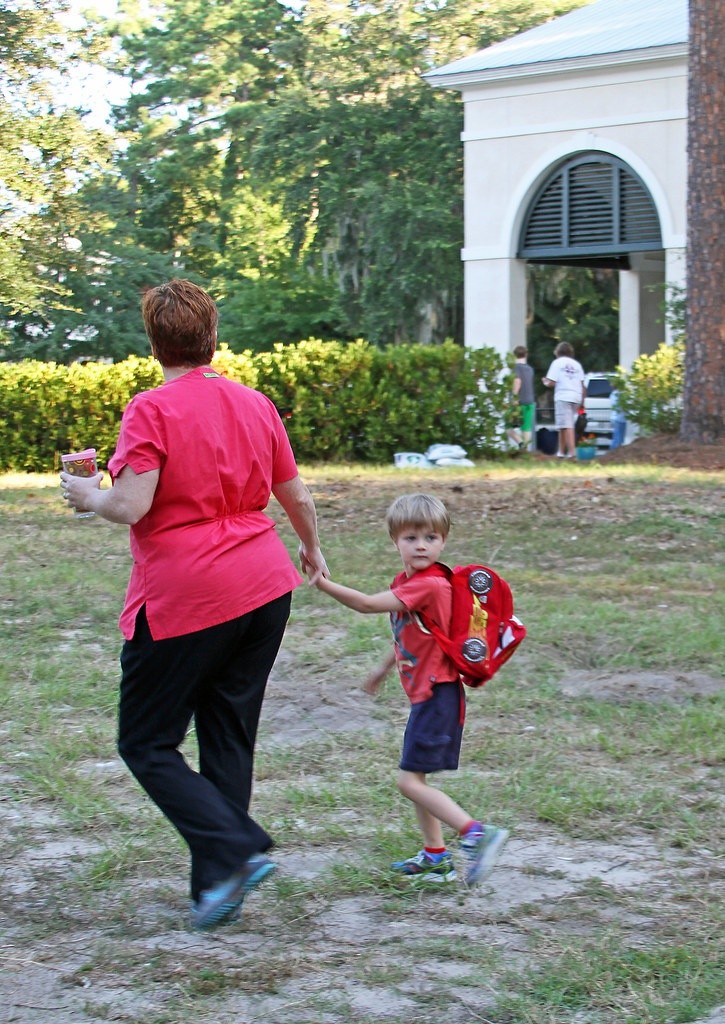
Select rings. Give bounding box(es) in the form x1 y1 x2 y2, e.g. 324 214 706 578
63 491 67 498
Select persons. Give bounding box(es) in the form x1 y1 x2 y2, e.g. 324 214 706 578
59 274 331 931
541 341 587 462
304 491 509 888
505 345 535 451
609 388 630 448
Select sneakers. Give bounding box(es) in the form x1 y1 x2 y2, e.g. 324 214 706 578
447 821 510 887
389 850 458 883
189 853 278 931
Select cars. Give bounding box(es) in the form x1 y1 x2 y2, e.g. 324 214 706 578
583 372 622 450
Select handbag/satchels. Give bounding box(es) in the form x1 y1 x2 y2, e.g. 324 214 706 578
575 408 587 435
511 404 524 428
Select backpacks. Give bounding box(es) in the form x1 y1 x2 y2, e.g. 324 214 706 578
410 561 526 688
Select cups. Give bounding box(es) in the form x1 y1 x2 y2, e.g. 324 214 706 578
61 448 100 519
